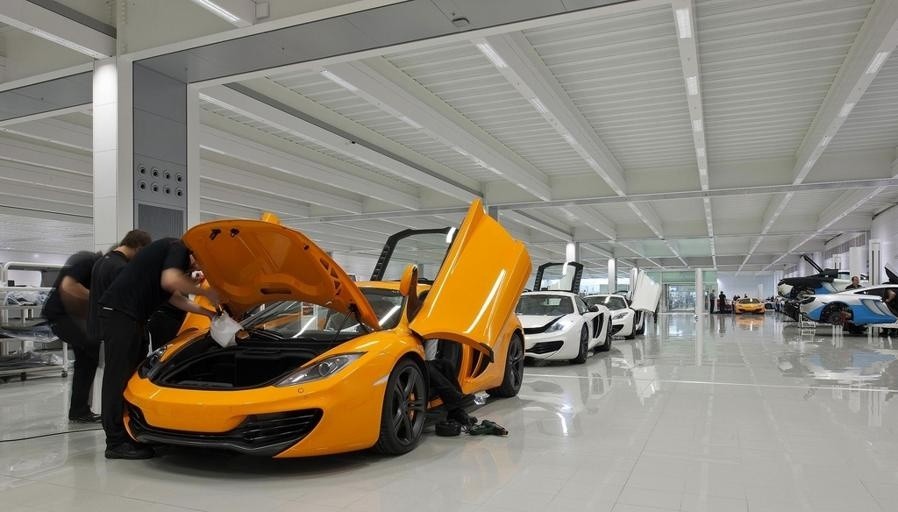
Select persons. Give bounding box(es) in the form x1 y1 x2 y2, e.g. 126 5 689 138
97 237 221 461
87 229 203 349
878 287 898 338
669 288 777 314
41 244 113 423
417 286 479 427
846 275 864 290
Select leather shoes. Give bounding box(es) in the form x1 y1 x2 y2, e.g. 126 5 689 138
70 412 102 422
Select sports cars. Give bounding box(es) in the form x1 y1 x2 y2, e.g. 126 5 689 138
764 253 897 337
732 298 765 314
122 195 526 458
513 262 612 365
579 270 662 340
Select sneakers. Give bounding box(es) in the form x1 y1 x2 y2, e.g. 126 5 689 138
447 407 478 424
447 394 475 411
105 441 158 458
